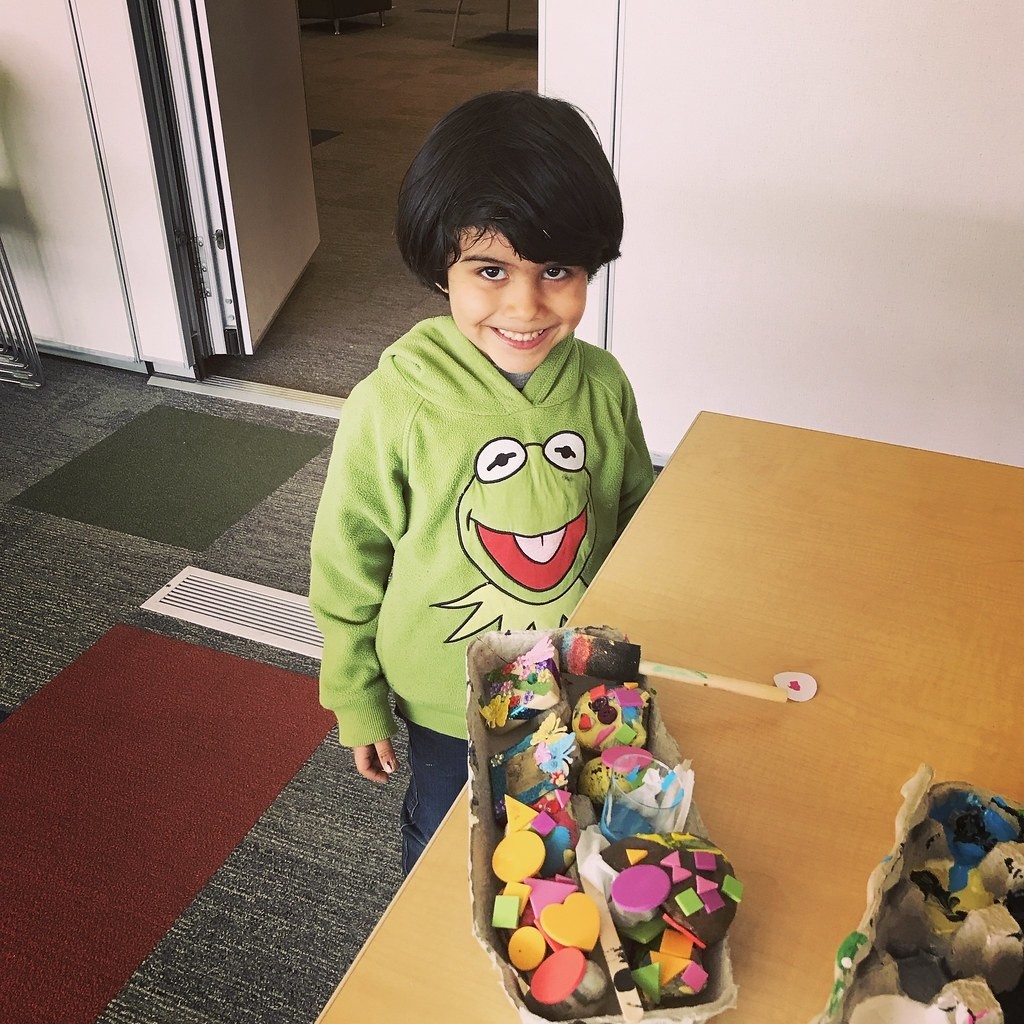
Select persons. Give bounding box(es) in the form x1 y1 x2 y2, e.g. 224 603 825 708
310 90 656 879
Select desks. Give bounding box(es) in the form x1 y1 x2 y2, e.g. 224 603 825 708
295 406 1024 1024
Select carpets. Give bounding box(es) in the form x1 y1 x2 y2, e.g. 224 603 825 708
1 620 337 1023
3 404 331 552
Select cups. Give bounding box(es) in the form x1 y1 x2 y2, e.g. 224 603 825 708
600 755 684 843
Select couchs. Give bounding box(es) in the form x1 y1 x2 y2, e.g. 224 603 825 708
300 0 391 35
